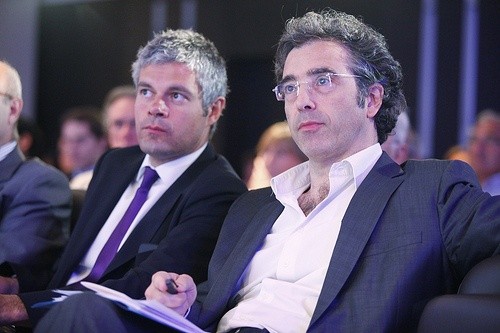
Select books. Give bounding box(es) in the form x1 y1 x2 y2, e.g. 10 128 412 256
31 280 207 333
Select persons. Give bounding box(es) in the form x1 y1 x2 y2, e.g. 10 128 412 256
31 8 500 333
0 61 500 333
0 27 248 333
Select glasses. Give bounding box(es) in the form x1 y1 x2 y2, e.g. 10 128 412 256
272 72 362 101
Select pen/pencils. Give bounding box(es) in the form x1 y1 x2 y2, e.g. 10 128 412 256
164 278 179 293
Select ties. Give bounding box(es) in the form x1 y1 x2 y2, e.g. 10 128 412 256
69 166 158 291
